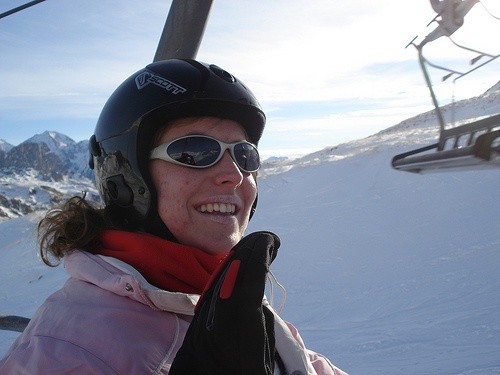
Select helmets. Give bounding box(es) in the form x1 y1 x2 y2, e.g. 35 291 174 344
85 56 268 238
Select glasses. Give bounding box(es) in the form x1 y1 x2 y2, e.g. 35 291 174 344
146 133 263 173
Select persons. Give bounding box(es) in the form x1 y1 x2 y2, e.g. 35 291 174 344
0 59 349 375
